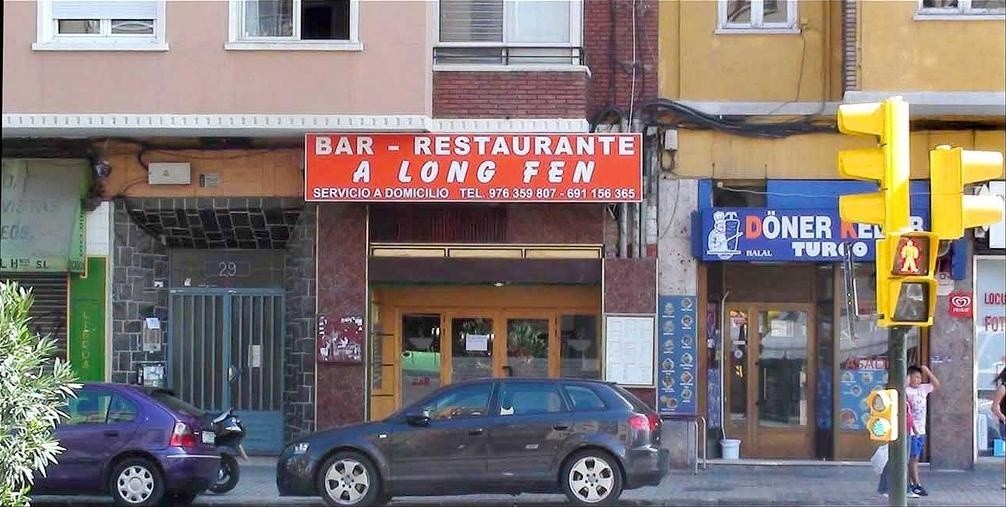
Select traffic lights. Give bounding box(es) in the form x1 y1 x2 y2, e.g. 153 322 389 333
838 100 910 231
885 232 938 325
929 144 1004 242
869 389 899 445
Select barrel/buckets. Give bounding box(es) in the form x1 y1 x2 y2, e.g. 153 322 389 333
720 438 742 459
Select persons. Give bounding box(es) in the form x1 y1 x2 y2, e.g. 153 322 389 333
990 365 1006 489
899 364 940 495
874 367 920 499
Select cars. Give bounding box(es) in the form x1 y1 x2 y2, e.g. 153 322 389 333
274 377 669 504
15 383 245 505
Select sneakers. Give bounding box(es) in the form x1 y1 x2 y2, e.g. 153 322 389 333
907 484 927 496
